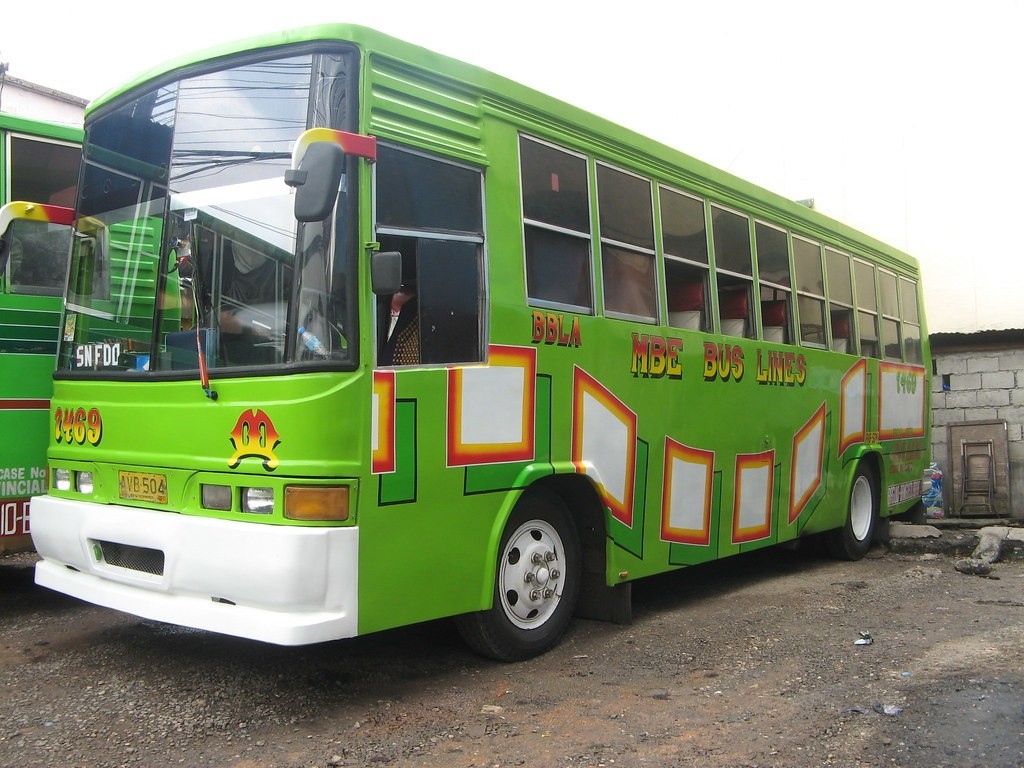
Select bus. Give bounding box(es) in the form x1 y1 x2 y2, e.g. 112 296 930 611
0 22 936 664
0 112 343 596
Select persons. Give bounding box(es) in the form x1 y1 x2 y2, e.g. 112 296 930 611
353 251 429 367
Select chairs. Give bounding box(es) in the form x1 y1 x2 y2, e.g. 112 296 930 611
667 270 851 353
382 290 449 365
